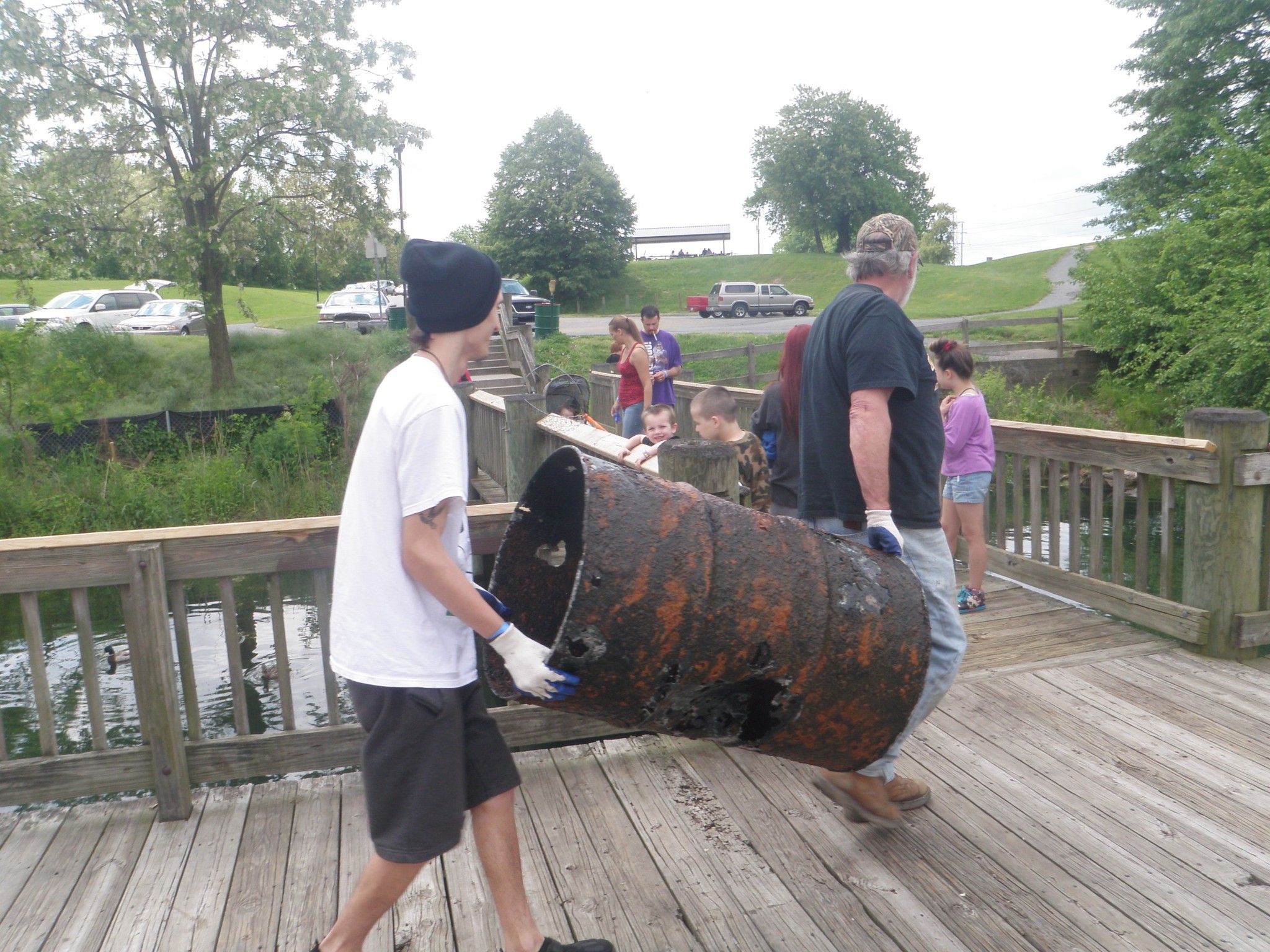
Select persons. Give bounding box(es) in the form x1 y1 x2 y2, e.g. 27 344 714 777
928 337 996 614
748 324 813 518
617 403 681 470
607 342 622 364
316 241 613 952
557 407 576 423
689 386 771 514
608 315 652 439
639 306 682 409
796 214 967 830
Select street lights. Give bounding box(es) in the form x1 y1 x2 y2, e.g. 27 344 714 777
391 134 408 234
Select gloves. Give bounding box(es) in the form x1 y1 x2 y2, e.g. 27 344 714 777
865 507 903 561
483 619 581 707
468 579 506 615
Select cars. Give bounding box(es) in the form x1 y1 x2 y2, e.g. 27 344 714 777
0 304 41 328
117 299 210 337
316 279 407 337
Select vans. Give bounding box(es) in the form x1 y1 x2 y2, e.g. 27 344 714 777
21 278 176 336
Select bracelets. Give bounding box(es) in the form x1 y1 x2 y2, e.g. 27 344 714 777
664 371 668 378
616 397 620 403
643 449 651 459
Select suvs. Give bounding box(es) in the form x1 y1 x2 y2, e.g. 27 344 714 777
498 279 550 330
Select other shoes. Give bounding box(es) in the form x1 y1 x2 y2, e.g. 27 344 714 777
526 931 620 951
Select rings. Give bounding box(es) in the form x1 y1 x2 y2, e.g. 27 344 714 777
660 379 662 381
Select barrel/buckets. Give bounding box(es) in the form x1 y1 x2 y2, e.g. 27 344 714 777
534 303 560 341
389 307 407 330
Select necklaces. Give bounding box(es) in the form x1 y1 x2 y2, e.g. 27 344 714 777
959 388 979 396
416 348 453 389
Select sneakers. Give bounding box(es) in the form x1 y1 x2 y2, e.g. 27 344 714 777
956 584 986 614
841 769 932 825
813 760 907 832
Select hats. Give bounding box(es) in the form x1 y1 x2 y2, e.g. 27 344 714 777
857 211 925 270
399 236 503 339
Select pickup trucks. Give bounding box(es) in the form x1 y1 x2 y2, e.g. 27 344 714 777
687 281 815 318
